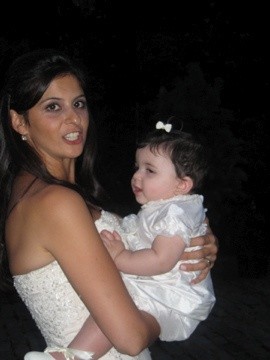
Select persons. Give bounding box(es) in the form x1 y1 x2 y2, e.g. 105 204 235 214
0 49 220 360
23 121 216 360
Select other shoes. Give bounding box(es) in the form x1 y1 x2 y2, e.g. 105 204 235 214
24 346 96 360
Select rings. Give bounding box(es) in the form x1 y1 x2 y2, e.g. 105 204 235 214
204 258 211 266
